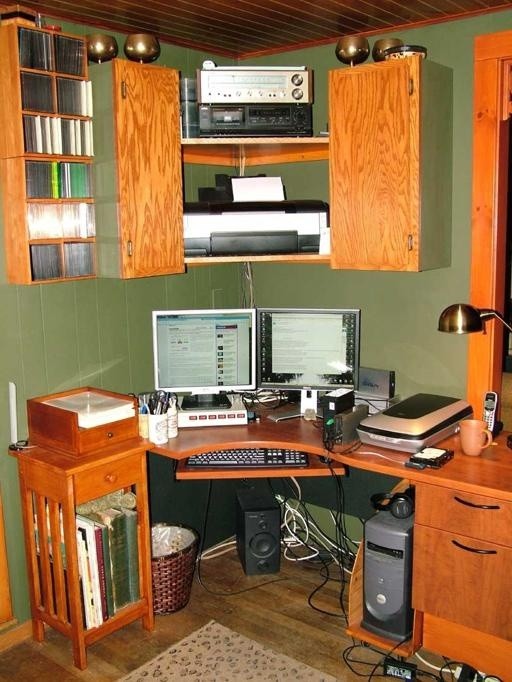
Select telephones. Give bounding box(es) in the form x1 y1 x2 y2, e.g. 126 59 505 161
481 391 497 435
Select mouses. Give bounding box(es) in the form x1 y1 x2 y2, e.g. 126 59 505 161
319 455 335 464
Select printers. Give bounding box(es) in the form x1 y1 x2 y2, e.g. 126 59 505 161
182 175 329 255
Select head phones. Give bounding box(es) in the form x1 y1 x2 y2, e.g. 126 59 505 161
370 491 413 519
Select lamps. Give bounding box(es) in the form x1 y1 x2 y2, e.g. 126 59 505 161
437 304 512 334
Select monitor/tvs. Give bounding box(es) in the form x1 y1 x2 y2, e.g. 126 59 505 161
152 308 256 410
257 308 360 424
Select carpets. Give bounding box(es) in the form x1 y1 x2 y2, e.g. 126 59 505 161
117 617 344 682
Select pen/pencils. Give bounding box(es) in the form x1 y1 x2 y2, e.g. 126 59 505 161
128 391 177 415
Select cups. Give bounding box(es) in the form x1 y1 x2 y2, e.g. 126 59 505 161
147 412 169 444
169 408 179 439
139 413 147 440
459 420 493 457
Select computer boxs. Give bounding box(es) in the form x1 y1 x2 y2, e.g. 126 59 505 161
236 484 281 577
361 367 395 399
360 507 413 643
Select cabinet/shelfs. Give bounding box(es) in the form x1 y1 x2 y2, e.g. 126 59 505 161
86 59 188 282
0 22 98 286
328 56 453 274
11 440 155 669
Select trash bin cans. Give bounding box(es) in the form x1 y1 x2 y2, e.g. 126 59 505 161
150 521 200 615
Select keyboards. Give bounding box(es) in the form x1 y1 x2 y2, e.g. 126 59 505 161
186 447 308 469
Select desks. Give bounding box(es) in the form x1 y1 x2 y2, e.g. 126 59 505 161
154 388 512 681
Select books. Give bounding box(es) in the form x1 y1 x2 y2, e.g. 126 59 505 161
28 203 96 240
54 34 83 74
39 391 135 430
26 161 93 198
21 72 54 113
33 505 138 629
63 242 97 278
31 244 62 279
23 114 94 157
18 28 52 71
56 76 93 117
